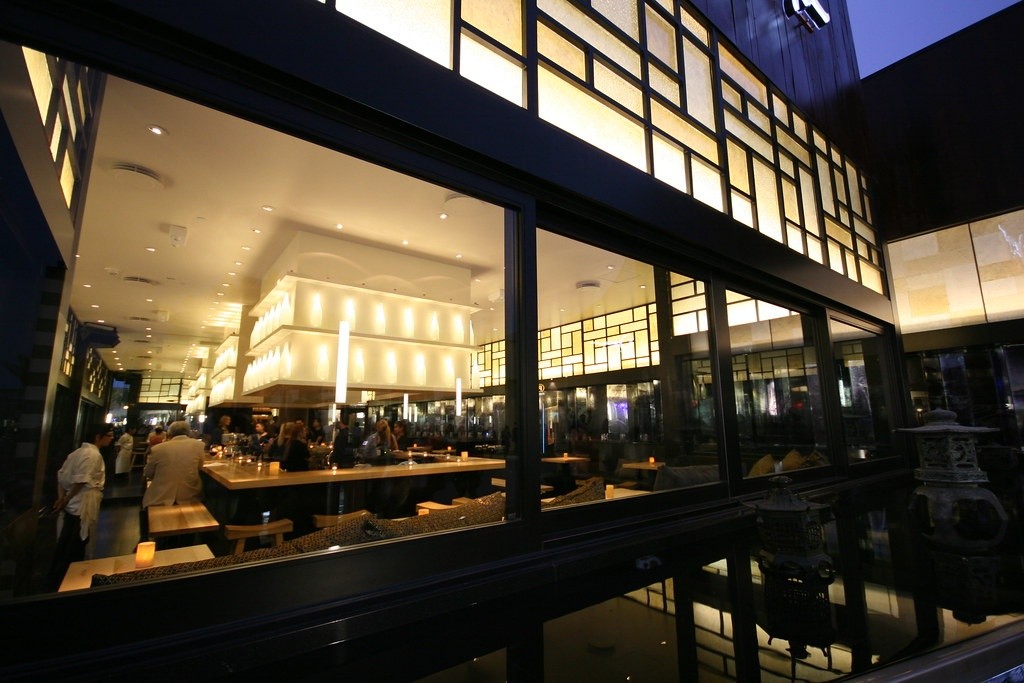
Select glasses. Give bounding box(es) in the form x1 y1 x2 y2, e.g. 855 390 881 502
103 433 112 438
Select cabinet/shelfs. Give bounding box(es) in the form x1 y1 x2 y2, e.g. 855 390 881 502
131 451 147 468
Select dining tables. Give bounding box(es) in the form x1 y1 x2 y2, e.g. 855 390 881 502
57 543 216 593
623 462 665 480
605 487 650 499
199 450 507 490
541 456 591 475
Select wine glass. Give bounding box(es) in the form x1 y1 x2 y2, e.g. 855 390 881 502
208 444 218 462
225 446 233 462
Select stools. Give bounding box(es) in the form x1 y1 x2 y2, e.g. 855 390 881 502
224 517 294 554
147 500 220 550
620 481 638 489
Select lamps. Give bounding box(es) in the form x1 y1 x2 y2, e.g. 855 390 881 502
649 456 654 463
606 485 614 499
461 451 468 461
564 453 568 459
269 461 280 475
135 541 156 569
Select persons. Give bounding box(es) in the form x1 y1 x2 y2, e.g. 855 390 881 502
504 420 519 457
146 414 407 473
114 421 138 484
48 421 115 606
141 419 206 548
